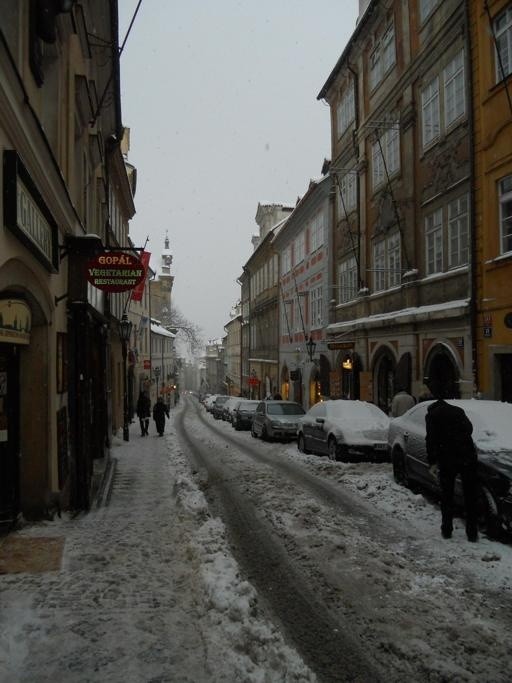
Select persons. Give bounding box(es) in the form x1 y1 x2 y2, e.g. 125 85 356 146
274 392 282 400
392 383 417 418
425 400 479 542
137 391 151 436
153 397 170 436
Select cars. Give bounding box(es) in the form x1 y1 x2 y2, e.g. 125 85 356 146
296 398 394 463
387 400 512 536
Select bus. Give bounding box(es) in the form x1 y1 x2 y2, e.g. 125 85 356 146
185 390 305 442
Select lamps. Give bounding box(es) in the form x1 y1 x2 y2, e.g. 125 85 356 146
306 336 322 364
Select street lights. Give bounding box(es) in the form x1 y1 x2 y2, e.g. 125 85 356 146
154 365 161 402
118 316 134 441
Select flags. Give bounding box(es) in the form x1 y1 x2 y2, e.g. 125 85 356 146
131 251 151 300
